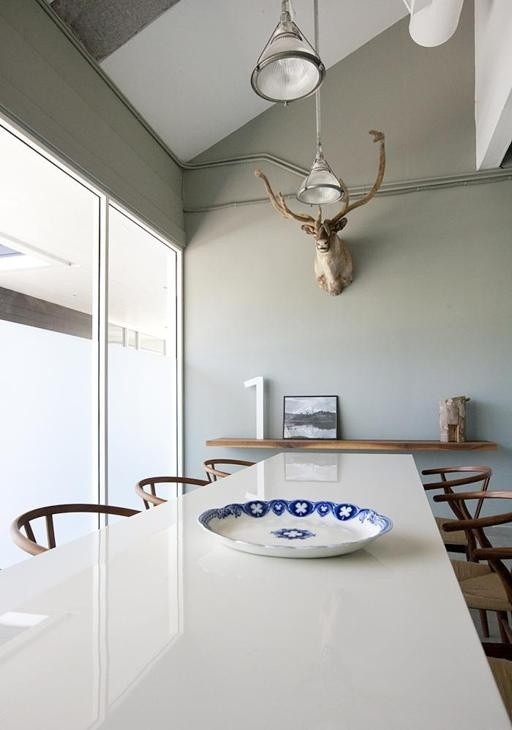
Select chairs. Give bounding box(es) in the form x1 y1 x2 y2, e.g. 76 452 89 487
11 459 256 557
422 466 512 724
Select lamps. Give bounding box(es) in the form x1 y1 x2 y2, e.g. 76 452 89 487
295 0 346 205
251 1 327 108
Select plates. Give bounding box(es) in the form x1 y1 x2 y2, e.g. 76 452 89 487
197 500 393 558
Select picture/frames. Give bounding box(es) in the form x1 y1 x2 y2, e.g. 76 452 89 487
282 395 339 440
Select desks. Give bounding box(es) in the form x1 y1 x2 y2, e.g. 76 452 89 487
1 454 512 730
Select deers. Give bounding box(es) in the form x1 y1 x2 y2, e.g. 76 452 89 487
254 129 385 296
